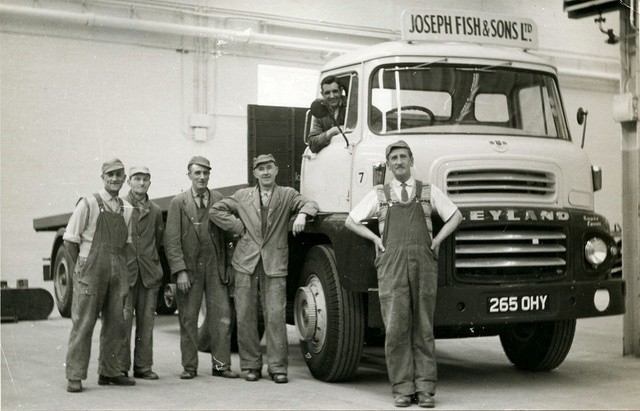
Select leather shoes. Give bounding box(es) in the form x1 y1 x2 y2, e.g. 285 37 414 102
99 372 136 385
416 391 434 407
134 370 158 379
246 370 262 380
394 394 412 407
67 379 81 392
180 370 197 379
213 367 239 378
271 373 288 383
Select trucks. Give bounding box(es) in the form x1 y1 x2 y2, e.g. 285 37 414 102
32 7 627 381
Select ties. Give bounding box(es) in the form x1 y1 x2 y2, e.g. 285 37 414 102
400 184 408 202
198 194 205 208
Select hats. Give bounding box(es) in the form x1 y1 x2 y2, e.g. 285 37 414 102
188 155 211 170
253 154 277 169
386 140 410 158
102 158 124 173
129 166 150 179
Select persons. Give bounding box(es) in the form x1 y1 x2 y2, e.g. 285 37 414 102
62 158 135 391
345 139 463 408
209 153 318 383
120 165 163 380
308 75 392 154
163 156 239 379
